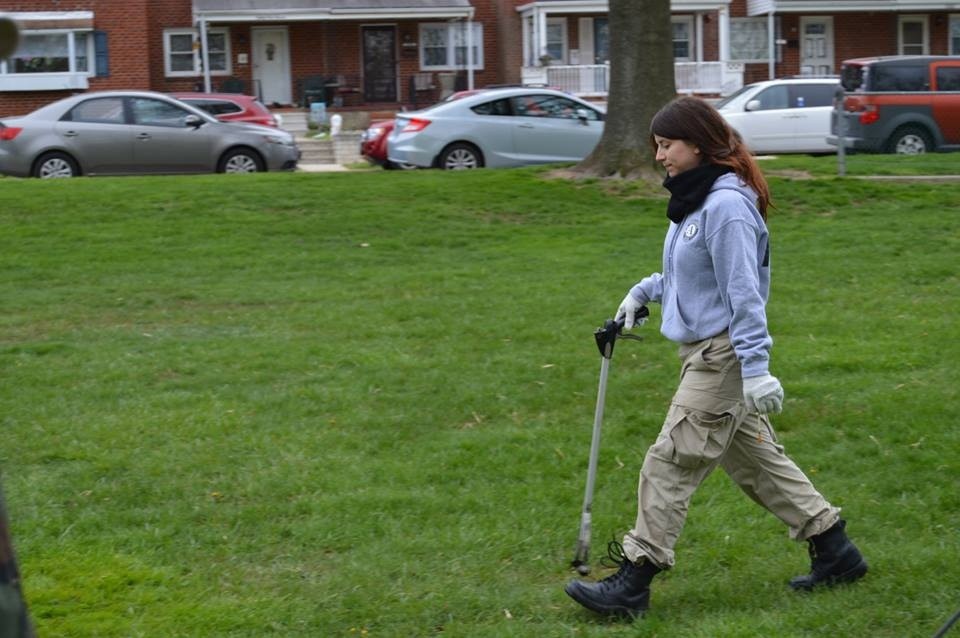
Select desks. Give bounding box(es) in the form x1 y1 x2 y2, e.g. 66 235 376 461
324 83 339 107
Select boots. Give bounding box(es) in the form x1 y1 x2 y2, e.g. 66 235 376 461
564 528 663 622
787 520 869 591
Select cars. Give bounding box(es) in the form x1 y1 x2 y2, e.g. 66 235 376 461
0 89 303 179
359 81 608 172
98 90 280 129
710 72 848 159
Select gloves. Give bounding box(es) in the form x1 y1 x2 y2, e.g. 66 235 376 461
742 374 784 415
614 292 649 331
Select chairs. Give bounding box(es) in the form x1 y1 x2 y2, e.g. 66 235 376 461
303 74 325 107
411 73 437 104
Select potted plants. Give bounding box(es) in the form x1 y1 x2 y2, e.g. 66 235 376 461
538 55 552 66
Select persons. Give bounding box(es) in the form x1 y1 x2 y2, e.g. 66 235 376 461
566 96 868 620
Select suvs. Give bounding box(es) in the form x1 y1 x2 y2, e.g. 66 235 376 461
825 55 960 157
